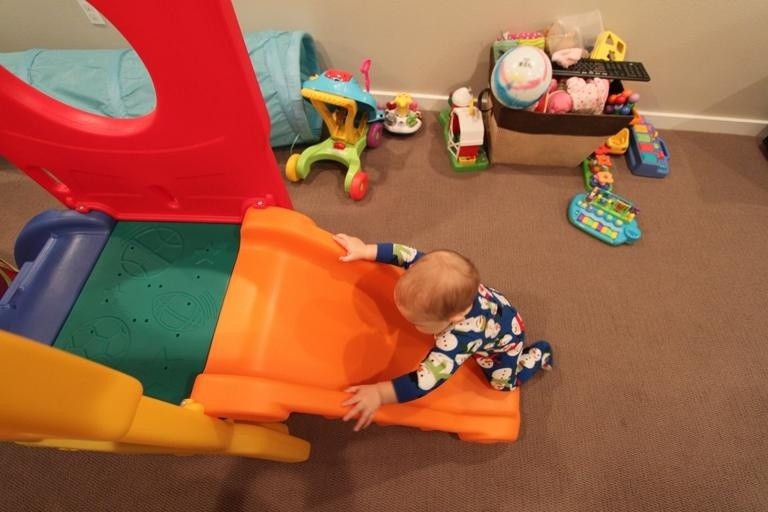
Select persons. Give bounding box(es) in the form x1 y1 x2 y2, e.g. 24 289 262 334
331 233 555 433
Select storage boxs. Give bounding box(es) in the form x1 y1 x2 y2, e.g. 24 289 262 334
485 45 633 167
